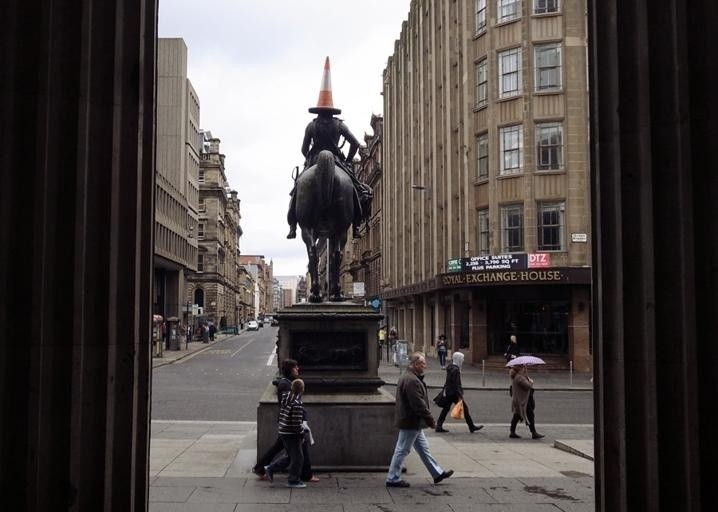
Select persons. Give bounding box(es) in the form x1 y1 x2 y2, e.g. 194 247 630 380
378 326 387 352
286 109 367 238
434 334 451 369
434 353 485 433
239 317 244 330
385 352 454 488
252 359 321 482
388 328 398 352
508 364 545 439
159 319 216 343
503 335 521 373
264 380 309 489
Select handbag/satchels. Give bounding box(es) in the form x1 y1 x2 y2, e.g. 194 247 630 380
510 386 534 401
433 390 447 408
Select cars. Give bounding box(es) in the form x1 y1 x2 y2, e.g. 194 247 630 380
247 320 259 331
258 318 269 327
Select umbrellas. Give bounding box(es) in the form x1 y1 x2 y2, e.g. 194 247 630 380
505 356 546 379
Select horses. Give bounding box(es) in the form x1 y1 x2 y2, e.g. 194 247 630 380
295 149 354 303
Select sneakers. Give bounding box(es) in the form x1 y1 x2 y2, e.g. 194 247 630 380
306 476 320 482
532 433 545 439
510 433 521 438
434 470 453 483
286 481 307 488
471 426 483 432
252 465 273 483
386 481 410 487
436 428 450 432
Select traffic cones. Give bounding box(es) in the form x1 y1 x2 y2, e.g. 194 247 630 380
309 56 341 114
